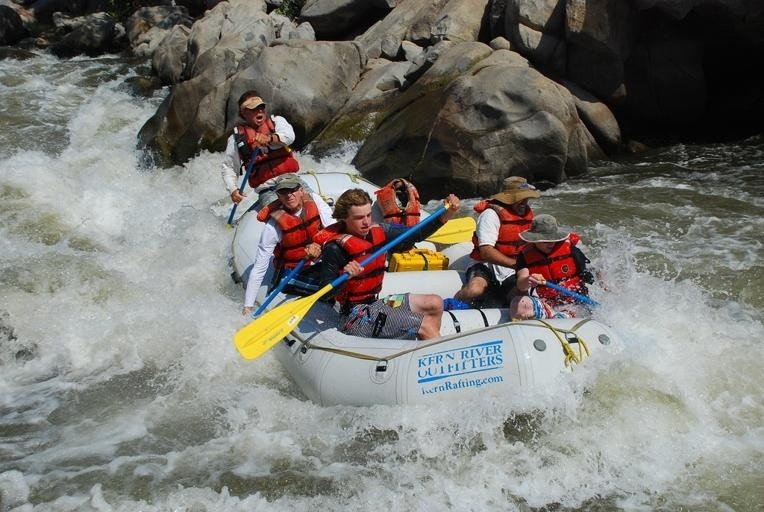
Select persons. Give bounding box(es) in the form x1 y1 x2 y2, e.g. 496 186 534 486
222 90 314 208
242 174 338 316
320 188 461 340
510 214 590 320
454 175 542 304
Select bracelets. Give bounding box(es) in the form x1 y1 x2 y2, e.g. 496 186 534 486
306 244 322 254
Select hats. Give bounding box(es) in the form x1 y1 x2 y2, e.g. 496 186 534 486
275 174 302 192
489 176 540 205
240 96 267 114
518 214 570 242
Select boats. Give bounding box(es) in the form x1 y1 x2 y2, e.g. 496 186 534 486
228 171 624 409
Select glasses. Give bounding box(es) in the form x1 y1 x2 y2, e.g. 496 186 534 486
277 184 300 195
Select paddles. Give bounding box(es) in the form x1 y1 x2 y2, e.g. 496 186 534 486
234 202 451 360
424 217 476 244
